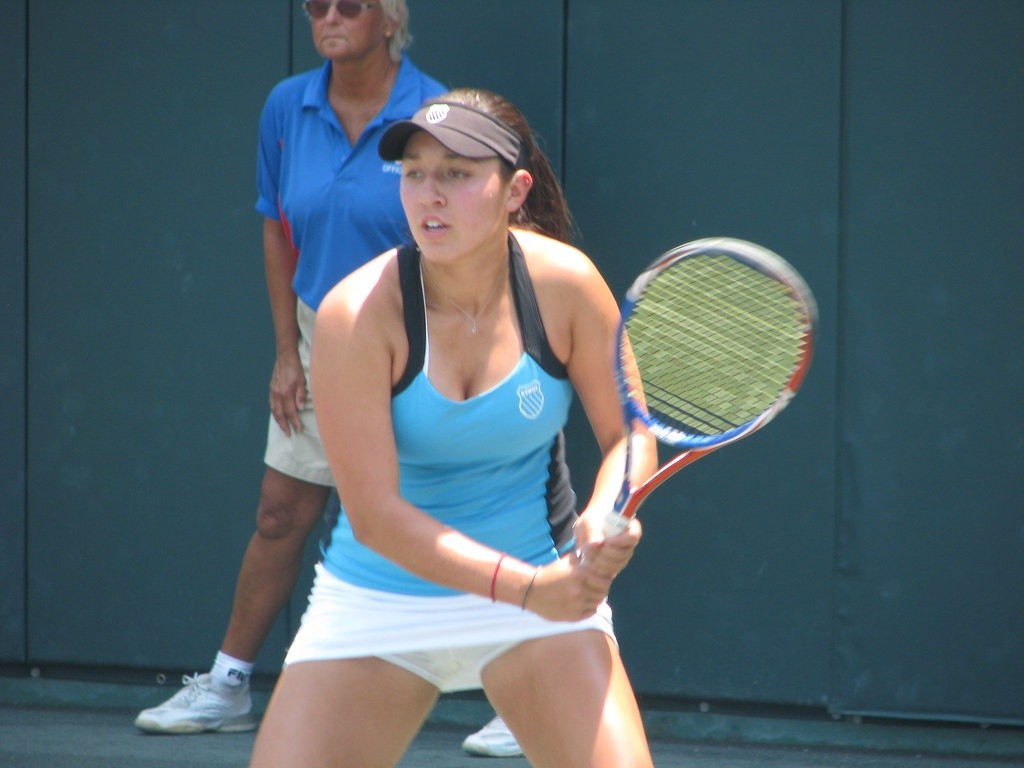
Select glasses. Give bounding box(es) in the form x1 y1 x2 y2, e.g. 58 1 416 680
305 1 380 17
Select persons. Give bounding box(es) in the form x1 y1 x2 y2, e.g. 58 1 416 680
132 0 529 758
244 79 670 768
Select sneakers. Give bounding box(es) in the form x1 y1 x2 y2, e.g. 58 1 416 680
463 714 523 756
136 675 256 731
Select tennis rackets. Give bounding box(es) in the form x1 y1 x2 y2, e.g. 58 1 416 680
596 237 821 540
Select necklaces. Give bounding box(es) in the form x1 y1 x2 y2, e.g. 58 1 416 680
419 249 512 335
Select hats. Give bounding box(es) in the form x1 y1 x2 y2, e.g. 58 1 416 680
378 103 523 167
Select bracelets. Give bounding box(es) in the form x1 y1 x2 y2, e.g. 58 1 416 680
521 561 547 613
489 550 510 603
